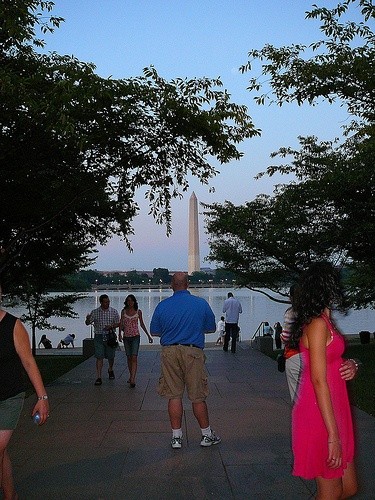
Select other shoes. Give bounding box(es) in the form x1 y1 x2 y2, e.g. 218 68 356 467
126 379 135 386
95 378 102 385
108 369 115 379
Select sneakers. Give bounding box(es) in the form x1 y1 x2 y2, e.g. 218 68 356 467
200 429 221 446
171 434 184 448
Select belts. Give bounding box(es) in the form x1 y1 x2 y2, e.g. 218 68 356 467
163 343 203 350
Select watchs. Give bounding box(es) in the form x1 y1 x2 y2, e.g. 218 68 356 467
38 395 48 401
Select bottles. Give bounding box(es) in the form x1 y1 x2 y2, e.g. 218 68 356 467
32 412 40 426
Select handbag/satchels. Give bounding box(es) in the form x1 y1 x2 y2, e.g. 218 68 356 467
278 350 285 372
108 326 118 348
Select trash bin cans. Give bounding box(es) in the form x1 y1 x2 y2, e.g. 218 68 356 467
358 328 373 348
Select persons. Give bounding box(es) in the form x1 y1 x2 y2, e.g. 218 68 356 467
117 294 154 388
57 333 75 349
281 259 359 500
1 293 54 500
150 272 221 447
215 293 243 353
38 334 52 349
263 322 283 351
84 295 119 386
